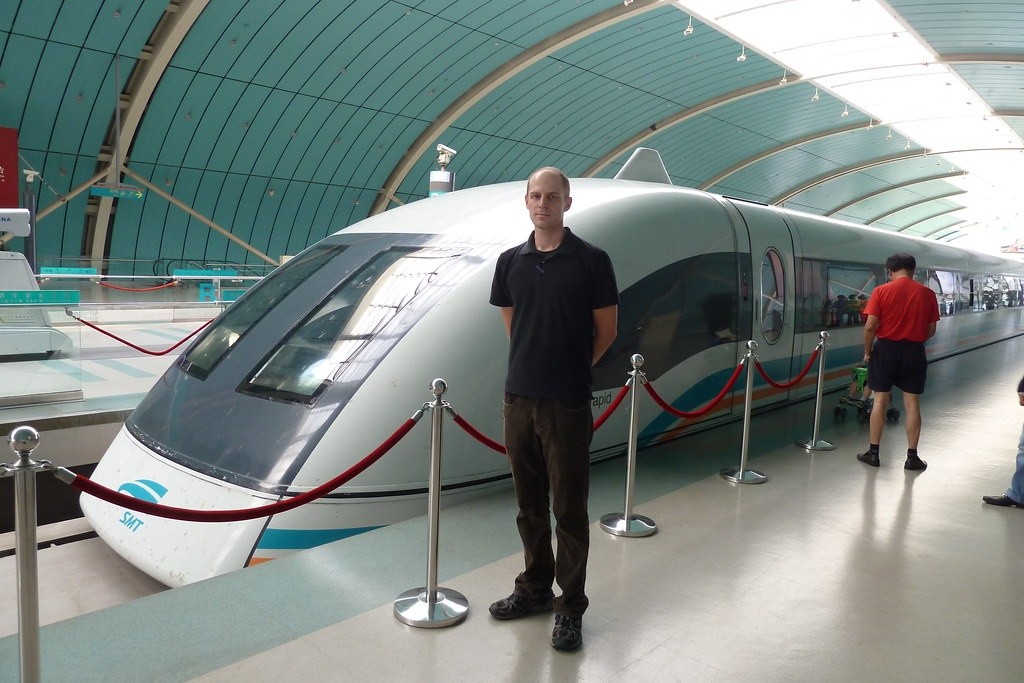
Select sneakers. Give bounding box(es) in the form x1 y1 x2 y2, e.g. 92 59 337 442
490 592 556 620
552 614 582 652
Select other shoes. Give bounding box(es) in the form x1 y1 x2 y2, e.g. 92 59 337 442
857 401 863 408
840 397 853 404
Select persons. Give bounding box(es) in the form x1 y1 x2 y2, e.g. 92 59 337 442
487 166 618 650
843 361 873 409
833 294 868 325
982 373 1024 507
856 252 941 470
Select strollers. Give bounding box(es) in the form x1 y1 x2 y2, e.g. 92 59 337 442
832 365 900 425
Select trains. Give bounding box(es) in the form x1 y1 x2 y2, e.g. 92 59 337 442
87 147 1023 591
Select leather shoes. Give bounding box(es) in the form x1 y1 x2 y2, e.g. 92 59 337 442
857 453 879 467
983 493 1024 510
905 458 928 471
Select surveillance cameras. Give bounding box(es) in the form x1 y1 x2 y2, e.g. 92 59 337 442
437 144 457 155
